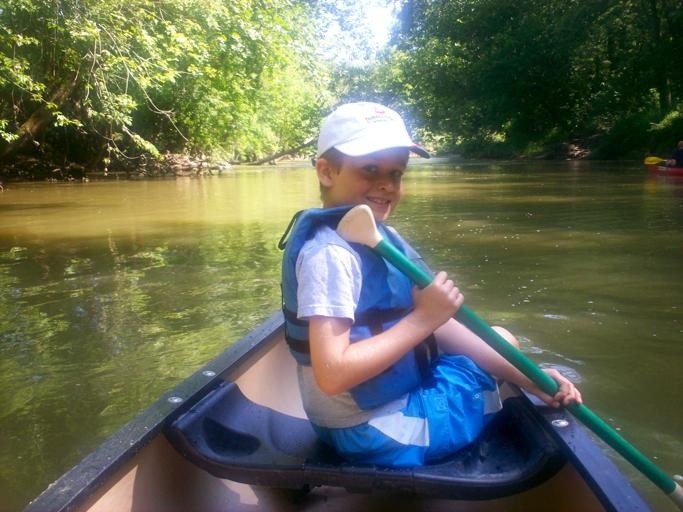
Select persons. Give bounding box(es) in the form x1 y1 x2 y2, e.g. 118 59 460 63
276 101 585 470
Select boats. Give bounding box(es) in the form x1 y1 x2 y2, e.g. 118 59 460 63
21 223 653 512
646 163 683 177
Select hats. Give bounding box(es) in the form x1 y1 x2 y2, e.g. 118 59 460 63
318 100 431 159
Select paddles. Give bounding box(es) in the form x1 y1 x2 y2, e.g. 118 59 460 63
645 157 668 165
